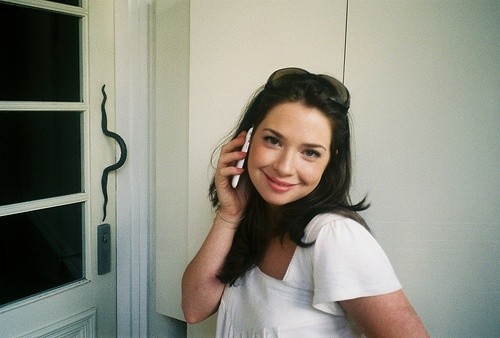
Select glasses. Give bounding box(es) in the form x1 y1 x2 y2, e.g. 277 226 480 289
265 67 350 114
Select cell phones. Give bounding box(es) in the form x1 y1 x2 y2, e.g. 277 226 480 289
231 127 253 188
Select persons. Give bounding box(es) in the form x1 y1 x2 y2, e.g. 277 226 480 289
180 67 432 337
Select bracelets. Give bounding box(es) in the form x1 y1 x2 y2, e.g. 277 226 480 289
216 209 245 223
